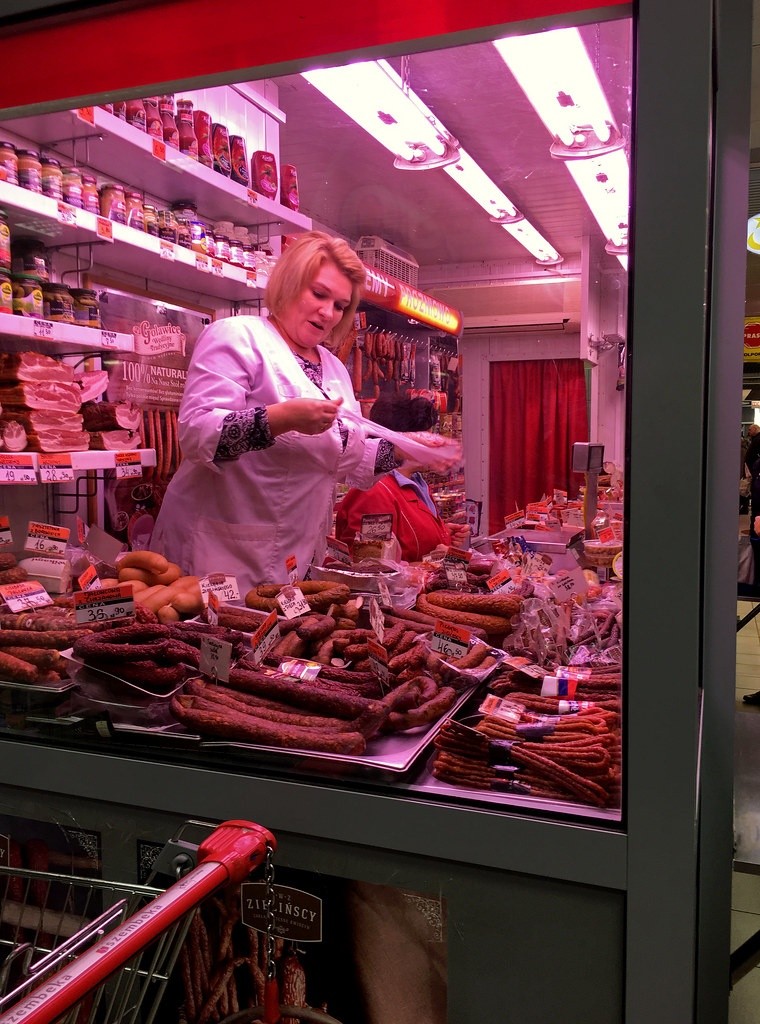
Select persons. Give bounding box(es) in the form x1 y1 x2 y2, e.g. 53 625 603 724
740 424 760 597
149 231 462 607
336 390 470 563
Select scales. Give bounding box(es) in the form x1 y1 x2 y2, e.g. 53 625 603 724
469 443 605 574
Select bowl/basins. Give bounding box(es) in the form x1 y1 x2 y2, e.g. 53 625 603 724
583 539 623 568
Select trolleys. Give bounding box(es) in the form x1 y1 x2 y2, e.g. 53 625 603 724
0 817 282 1024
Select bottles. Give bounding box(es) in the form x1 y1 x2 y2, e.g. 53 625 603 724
212 122 231 179
251 150 278 201
229 135 249 188
281 164 299 212
194 110 213 170
159 93 179 153
174 99 198 160
142 95 163 142
98 102 114 114
114 101 126 122
126 99 146 134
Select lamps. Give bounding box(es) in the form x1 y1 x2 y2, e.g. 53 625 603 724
301 26 629 270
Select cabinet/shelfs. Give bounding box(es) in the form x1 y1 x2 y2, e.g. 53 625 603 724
0 104 312 468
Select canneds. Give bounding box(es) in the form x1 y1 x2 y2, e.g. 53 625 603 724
0 141 277 276
0 210 101 329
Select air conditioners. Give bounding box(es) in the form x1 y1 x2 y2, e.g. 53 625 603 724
422 278 580 336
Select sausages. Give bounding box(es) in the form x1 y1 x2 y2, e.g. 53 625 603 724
136 409 182 481
352 331 406 398
0 551 622 810
440 356 463 414
180 886 307 1024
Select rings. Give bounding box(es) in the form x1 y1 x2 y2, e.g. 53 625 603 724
321 423 328 430
